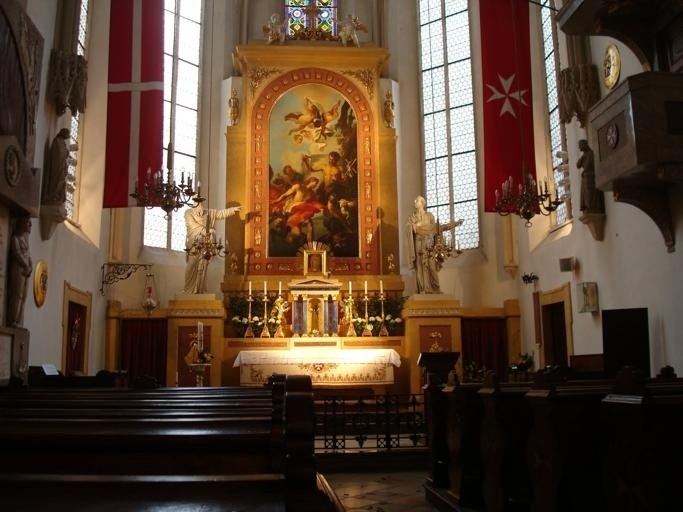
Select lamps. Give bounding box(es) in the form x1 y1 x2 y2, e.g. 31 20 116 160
129 0 206 222
493 0 565 229
185 0 230 262
418 0 462 270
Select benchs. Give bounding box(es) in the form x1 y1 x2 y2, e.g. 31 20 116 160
0 374 318 512
422 377 683 512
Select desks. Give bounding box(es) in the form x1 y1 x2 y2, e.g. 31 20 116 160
232 347 402 387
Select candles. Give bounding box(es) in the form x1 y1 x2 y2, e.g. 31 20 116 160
263 281 268 295
348 280 352 295
364 280 367 294
279 281 281 295
248 280 251 295
379 279 383 294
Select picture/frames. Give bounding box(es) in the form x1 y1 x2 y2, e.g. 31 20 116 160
61 279 93 377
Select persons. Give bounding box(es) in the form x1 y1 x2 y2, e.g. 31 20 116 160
268 148 357 255
44 128 70 204
6 214 33 328
182 195 243 295
575 139 597 211
406 195 464 295
